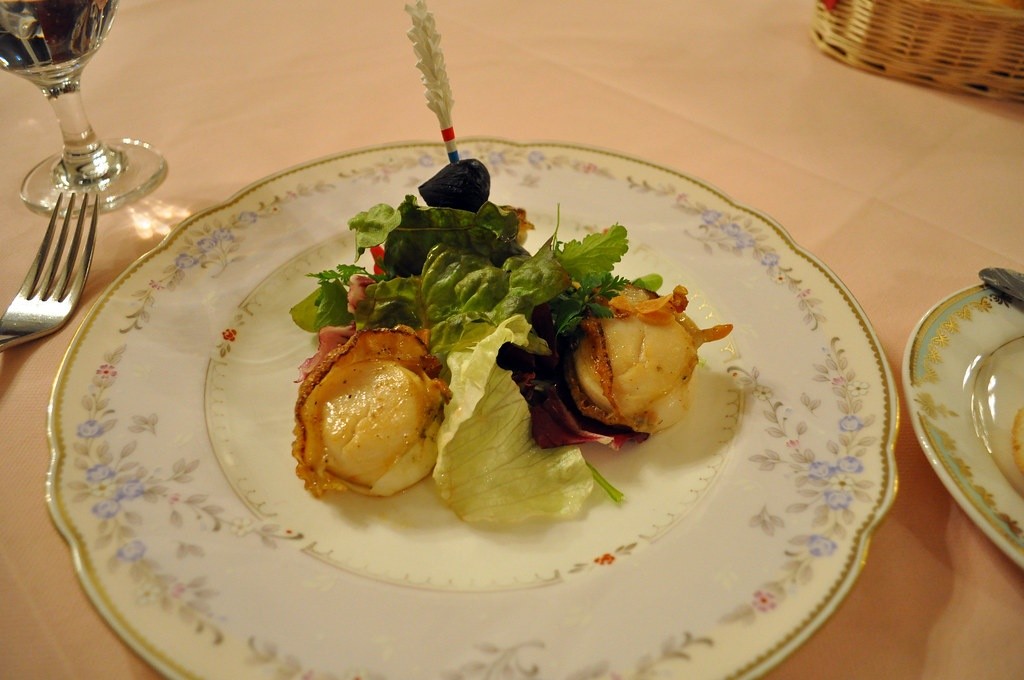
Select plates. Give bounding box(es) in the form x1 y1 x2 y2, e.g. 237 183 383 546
44 139 899 680
902 273 1024 568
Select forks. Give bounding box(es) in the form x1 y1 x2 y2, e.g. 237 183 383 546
1 193 99 353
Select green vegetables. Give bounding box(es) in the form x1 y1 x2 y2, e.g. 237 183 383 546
285 159 662 527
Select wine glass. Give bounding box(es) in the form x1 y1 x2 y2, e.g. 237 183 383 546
1 0 168 217
